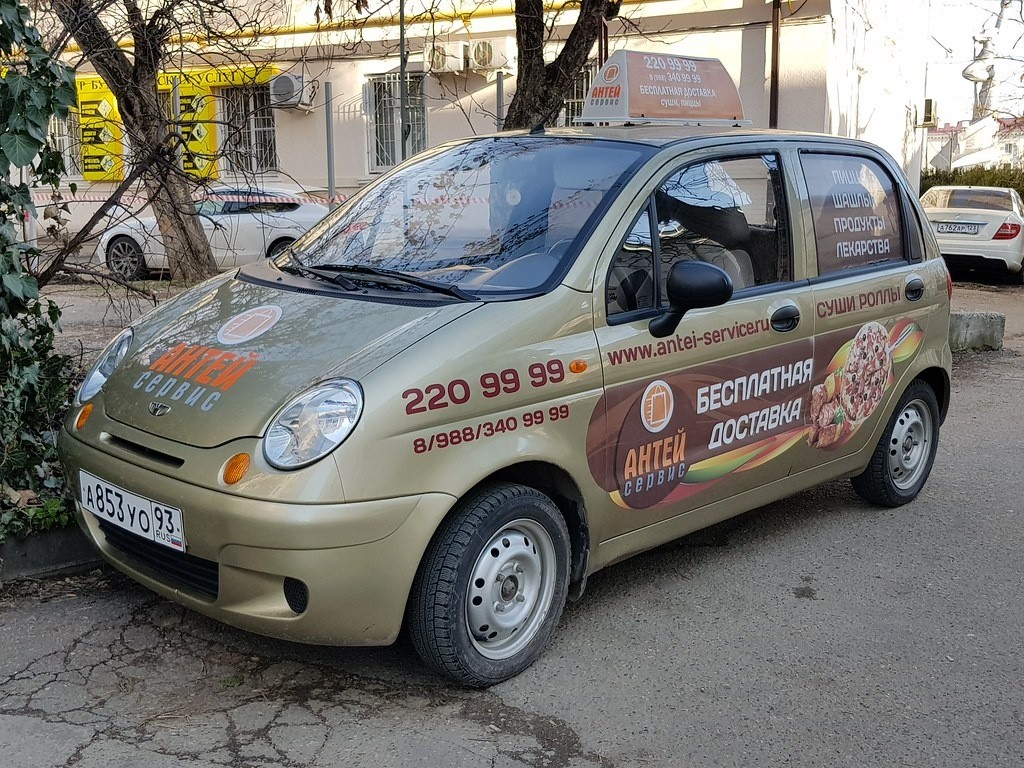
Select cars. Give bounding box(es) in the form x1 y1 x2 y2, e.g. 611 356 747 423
57 49 952 688
97 183 359 281
918 186 1024 284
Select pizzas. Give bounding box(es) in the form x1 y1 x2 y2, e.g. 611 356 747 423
841 321 890 422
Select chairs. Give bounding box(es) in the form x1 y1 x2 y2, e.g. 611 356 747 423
678 192 753 291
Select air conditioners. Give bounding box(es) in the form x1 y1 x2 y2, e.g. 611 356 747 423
270 73 312 106
423 41 469 72
467 35 519 69
923 98 937 127
